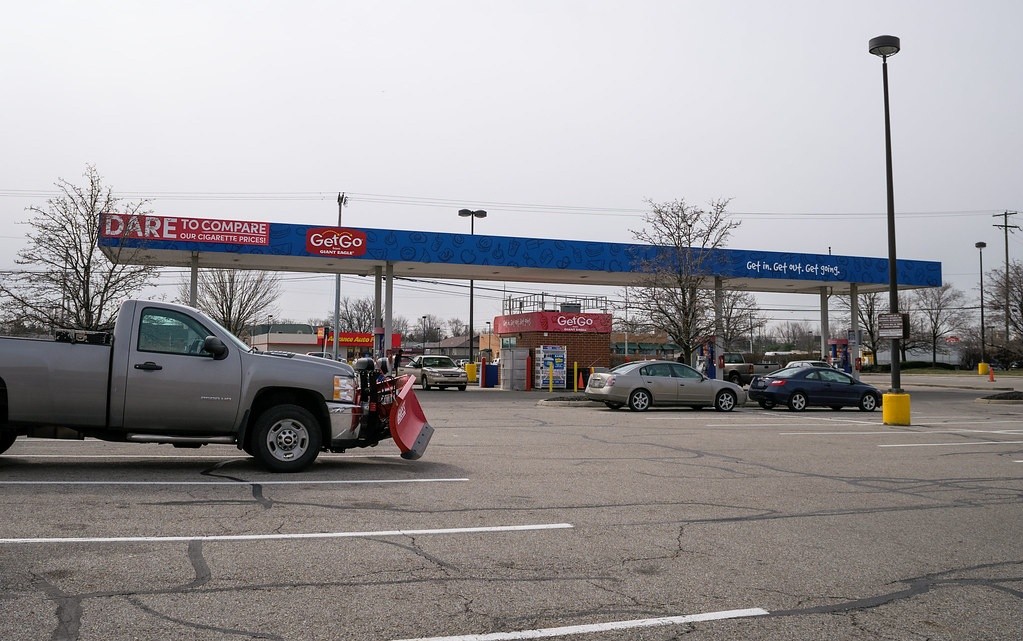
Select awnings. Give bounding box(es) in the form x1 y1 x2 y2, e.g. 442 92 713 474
610 342 682 351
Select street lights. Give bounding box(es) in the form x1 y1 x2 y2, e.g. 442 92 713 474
975 242 985 362
268 315 272 323
485 322 490 365
807 331 812 354
422 316 426 353
868 35 909 395
437 328 441 355
458 209 487 363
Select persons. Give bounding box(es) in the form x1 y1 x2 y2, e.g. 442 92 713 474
677 354 684 363
822 355 828 362
394 349 403 377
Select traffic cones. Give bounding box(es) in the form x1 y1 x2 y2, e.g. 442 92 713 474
988 368 996 382
577 372 585 391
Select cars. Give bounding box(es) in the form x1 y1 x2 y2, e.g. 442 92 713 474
748 367 882 412
308 351 346 363
785 361 852 380
394 354 467 391
585 360 746 413
456 358 469 365
492 358 501 364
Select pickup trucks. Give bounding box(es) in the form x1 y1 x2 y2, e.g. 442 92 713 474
710 350 781 385
0 298 436 474
